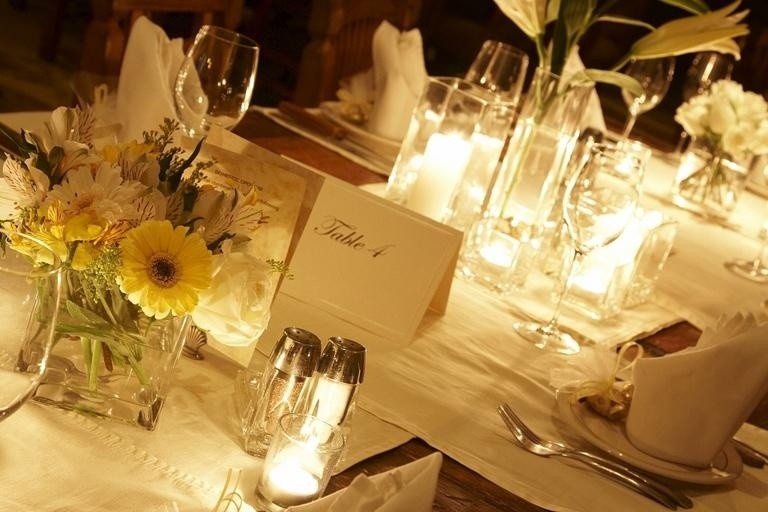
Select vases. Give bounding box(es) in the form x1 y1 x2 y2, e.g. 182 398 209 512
499 68 597 216
13 267 192 433
679 143 741 209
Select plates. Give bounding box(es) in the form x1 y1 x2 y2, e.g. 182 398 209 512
555 378 745 485
321 97 404 163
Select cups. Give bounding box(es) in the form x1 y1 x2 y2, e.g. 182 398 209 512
253 414 346 512
0 232 63 422
464 40 530 129
175 24 261 143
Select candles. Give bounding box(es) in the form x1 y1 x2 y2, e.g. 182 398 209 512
406 131 467 224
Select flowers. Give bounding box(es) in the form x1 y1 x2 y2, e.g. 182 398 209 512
493 0 749 212
676 79 766 201
2 87 274 393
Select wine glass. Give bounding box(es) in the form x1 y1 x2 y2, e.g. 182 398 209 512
660 52 736 168
723 242 768 283
595 41 679 158
511 140 653 358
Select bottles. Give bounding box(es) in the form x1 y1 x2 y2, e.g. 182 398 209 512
294 335 364 433
238 326 322 456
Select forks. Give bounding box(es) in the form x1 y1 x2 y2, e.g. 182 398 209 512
496 402 694 510
